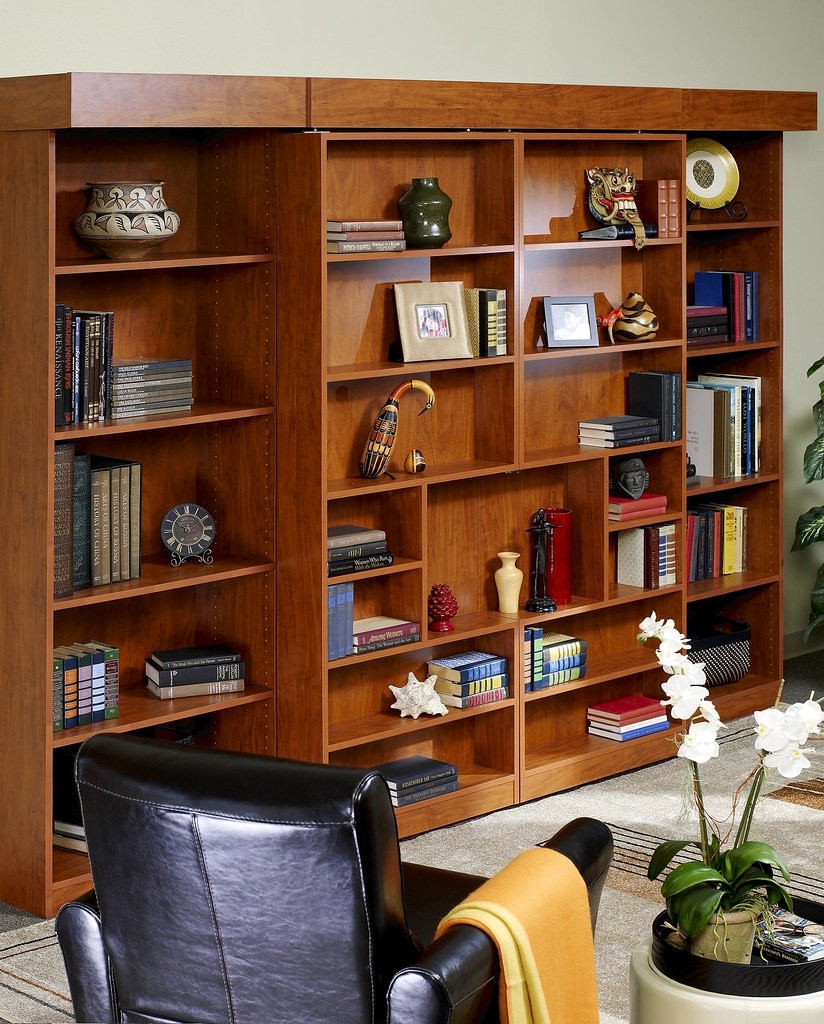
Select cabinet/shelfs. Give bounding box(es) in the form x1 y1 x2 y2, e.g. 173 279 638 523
0 72 818 918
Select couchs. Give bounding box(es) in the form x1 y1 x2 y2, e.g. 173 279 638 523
55 731 614 1024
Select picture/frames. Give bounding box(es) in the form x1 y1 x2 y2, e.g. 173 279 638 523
393 282 475 362
543 297 600 348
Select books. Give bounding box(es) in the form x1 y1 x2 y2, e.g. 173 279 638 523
687 268 763 347
638 179 684 241
368 754 458 808
580 225 658 243
576 369 683 448
49 799 93 857
142 645 247 699
607 495 747 588
56 303 196 426
323 526 423 665
586 692 672 744
684 371 765 483
428 650 509 708
464 288 507 361
53 447 143 600
520 623 586 692
52 641 122 729
325 219 410 257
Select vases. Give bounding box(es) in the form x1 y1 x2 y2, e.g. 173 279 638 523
690 893 764 965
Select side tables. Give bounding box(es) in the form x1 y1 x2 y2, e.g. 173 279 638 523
628 936 824 1024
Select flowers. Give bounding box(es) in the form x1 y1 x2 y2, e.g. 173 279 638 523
636 608 824 965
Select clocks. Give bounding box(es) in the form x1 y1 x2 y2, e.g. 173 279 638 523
161 502 213 567
